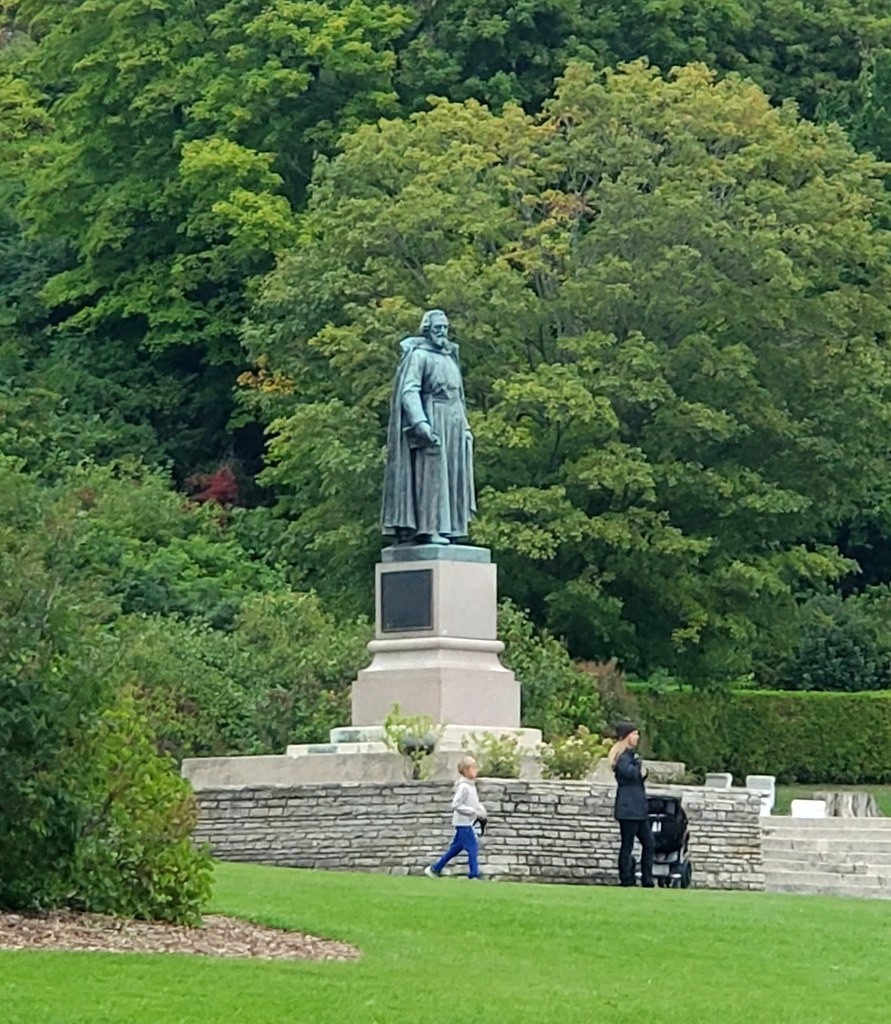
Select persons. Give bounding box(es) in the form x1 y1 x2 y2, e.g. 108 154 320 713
379 310 477 543
424 757 488 882
608 724 660 888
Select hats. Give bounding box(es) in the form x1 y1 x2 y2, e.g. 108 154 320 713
616 724 637 740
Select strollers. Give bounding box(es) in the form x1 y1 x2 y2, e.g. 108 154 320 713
640 792 694 890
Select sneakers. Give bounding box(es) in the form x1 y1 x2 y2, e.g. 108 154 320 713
424 865 439 879
